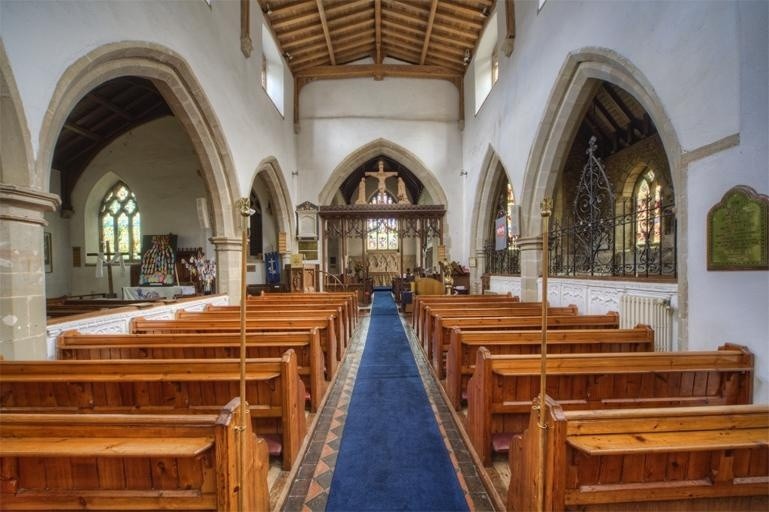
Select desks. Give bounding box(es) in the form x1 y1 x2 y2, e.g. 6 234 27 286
122 286 196 300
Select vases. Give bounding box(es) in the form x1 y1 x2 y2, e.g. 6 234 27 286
203 279 211 294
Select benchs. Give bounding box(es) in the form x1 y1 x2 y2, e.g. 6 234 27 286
0 291 360 512
413 291 769 512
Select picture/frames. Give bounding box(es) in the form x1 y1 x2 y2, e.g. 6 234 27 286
468 257 477 268
707 185 769 270
44 232 53 273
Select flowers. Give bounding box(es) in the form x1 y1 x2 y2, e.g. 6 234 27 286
181 256 217 281
